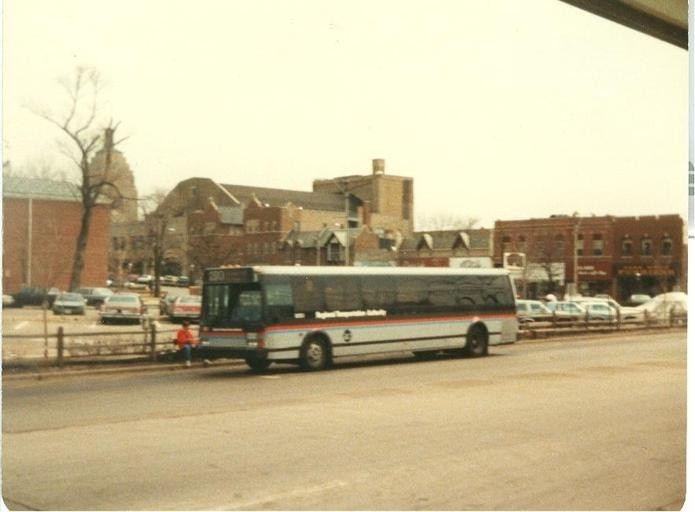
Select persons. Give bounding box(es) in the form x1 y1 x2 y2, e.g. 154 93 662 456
178 320 212 367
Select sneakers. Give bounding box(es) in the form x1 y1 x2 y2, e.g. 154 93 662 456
185 361 191 367
204 360 212 365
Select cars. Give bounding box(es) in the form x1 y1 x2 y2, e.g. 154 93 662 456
516 293 687 321
2 274 202 324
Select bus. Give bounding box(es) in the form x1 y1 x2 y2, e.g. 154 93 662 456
198 266 519 371
